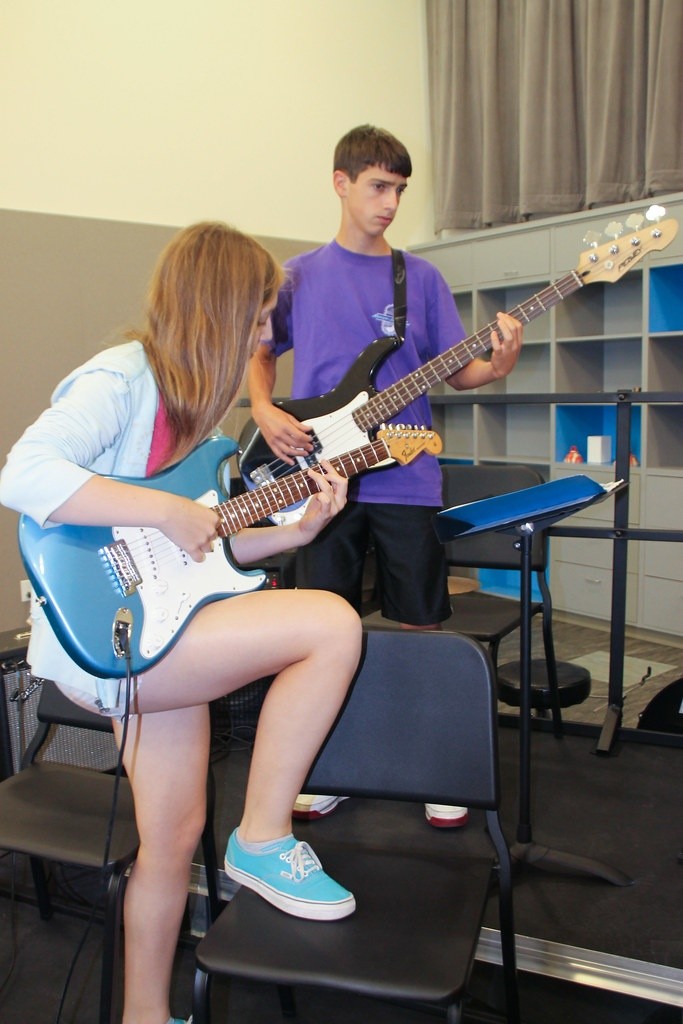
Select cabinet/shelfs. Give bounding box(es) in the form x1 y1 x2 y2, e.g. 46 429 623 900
405 193 683 637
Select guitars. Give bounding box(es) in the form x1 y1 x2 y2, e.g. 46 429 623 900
17 422 445 679
235 207 680 531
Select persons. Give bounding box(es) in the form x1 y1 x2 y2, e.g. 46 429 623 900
0 221 358 1024
247 125 524 830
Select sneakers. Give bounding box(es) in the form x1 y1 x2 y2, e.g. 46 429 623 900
224 827 356 921
292 794 349 818
166 1015 200 1024
424 803 468 828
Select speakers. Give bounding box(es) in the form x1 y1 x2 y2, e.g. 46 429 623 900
0 625 127 778
208 554 298 741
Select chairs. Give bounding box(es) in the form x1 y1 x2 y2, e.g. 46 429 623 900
0 679 220 1024
195 631 517 1024
358 466 562 739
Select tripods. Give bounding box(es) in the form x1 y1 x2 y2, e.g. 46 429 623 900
443 477 636 901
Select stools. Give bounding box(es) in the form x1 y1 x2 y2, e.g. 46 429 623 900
495 658 592 719
